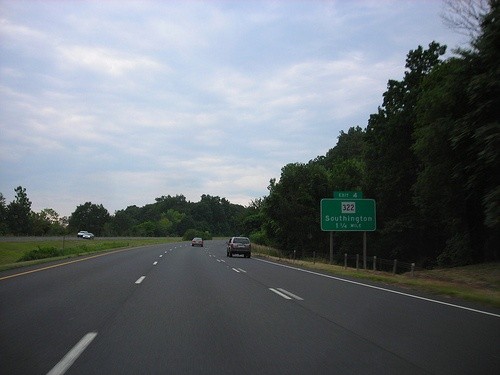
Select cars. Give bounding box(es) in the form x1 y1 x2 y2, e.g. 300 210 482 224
226 237 251 258
76 231 87 237
83 233 94 239
191 237 202 246
